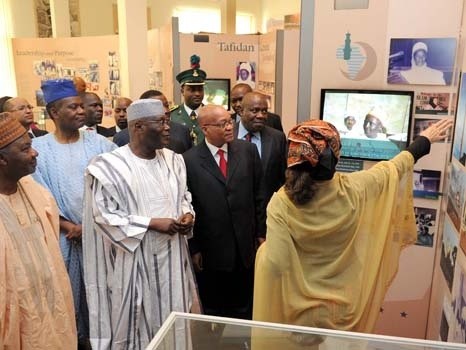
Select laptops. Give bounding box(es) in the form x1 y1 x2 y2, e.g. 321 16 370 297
320 89 415 173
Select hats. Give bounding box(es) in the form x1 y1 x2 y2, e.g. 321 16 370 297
40 78 78 105
176 68 207 86
125 99 165 122
0 111 27 149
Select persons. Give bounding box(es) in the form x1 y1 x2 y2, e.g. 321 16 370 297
80 100 200 350
181 105 267 339
421 96 445 110
391 40 445 84
0 112 77 350
256 117 454 349
413 178 426 190
338 106 397 141
0 70 287 350
413 207 434 245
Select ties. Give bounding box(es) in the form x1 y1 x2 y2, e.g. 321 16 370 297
244 134 254 142
190 111 197 120
216 149 228 179
28 133 34 139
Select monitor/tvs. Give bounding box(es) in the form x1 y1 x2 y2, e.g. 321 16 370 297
201 79 231 110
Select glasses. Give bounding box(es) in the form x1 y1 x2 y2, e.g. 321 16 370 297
10 106 33 113
205 118 234 128
144 119 169 126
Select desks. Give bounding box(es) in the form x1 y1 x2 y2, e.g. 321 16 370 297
143 311 466 350
